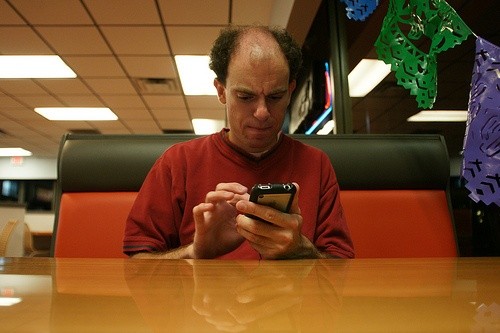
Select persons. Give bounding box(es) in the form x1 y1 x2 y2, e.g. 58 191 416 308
125 259 355 333
123 23 354 259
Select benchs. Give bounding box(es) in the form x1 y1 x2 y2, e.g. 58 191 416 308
47 133 461 258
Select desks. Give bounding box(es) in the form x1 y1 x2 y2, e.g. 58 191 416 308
0 255 500 333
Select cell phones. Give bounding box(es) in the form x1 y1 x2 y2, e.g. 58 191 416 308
246 183 297 224
